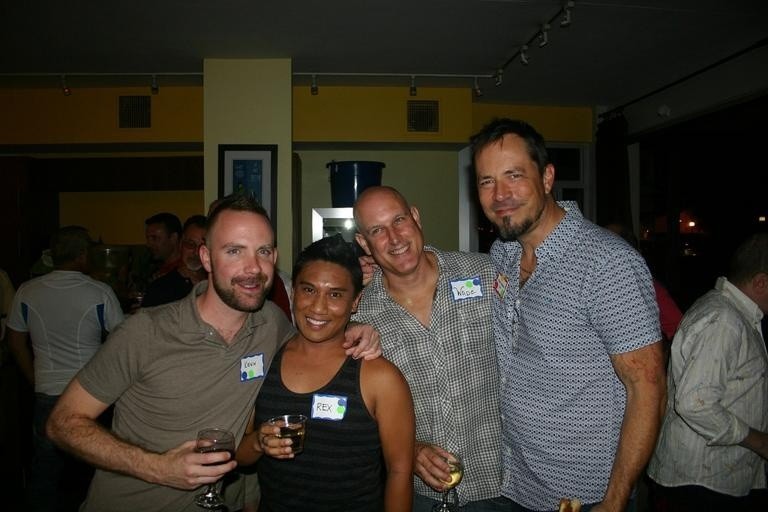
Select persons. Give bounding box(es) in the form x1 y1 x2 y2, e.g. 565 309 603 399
2 191 382 512
1 118 767 512
601 216 767 511
358 119 667 512
233 232 415 511
352 186 501 511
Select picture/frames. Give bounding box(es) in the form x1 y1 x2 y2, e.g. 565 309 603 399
217 141 279 251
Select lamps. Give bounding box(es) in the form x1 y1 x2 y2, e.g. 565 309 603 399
308 74 319 97
473 3 572 99
57 72 72 98
149 75 160 97
409 74 419 97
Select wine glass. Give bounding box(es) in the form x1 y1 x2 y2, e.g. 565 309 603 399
132 282 146 305
192 428 235 508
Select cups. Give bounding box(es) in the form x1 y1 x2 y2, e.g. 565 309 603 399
430 454 463 512
269 415 307 455
